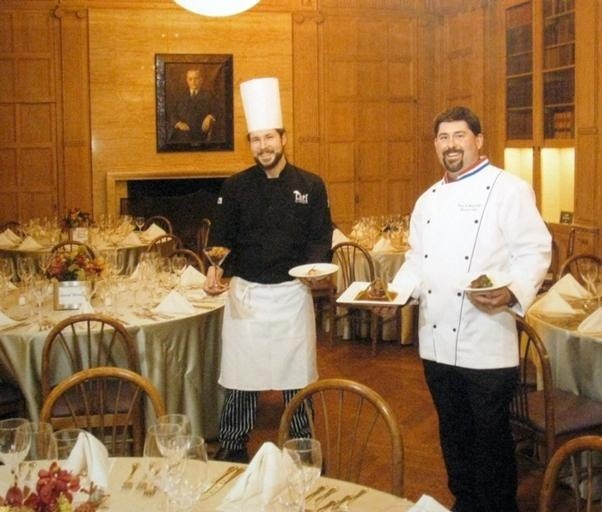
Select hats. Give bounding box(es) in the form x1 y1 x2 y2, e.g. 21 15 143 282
239 77 283 132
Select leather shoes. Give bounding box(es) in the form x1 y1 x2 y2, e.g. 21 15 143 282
213 448 248 462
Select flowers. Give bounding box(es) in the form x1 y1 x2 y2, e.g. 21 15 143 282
36 247 105 280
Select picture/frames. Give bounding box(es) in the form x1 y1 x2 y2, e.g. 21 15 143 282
154 52 235 153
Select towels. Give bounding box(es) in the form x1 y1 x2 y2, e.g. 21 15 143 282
173 266 210 284
226 440 298 505
54 431 111 491
130 263 153 280
2 309 24 333
399 495 453 510
529 275 602 331
333 222 411 253
151 291 196 314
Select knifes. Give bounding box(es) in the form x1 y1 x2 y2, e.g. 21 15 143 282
301 486 366 512
200 465 245 498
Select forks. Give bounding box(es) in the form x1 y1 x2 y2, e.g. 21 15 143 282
122 461 161 497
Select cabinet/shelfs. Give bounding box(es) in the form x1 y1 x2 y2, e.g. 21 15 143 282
503 2 575 143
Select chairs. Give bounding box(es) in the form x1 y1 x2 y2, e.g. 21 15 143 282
558 252 601 282
506 317 601 510
2 209 215 272
278 378 404 503
40 311 147 456
325 246 403 357
38 366 168 459
536 435 600 511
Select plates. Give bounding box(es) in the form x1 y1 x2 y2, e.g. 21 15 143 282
337 282 418 306
459 277 513 291
289 263 340 280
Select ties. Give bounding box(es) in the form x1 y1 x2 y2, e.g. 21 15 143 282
193 90 195 98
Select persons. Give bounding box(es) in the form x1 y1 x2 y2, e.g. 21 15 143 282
203 129 332 476
168 67 224 141
367 106 552 512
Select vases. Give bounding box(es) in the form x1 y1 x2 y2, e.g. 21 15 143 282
55 279 89 309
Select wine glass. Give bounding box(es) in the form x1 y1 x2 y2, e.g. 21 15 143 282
0 419 93 512
351 211 413 251
15 215 145 247
204 247 231 292
0 258 152 330
282 438 323 512
153 257 190 292
580 263 602 314
142 414 208 512
